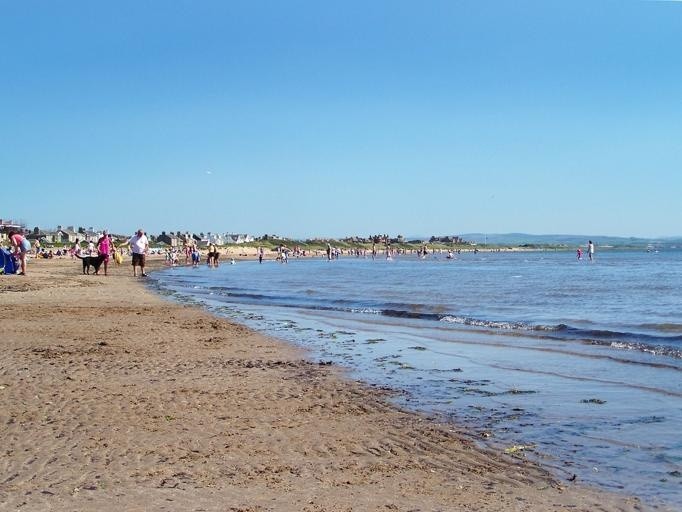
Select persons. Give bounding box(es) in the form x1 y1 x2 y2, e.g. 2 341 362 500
586 240 594 260
574 248 582 260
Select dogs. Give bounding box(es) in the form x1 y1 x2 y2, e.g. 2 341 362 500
75 253 109 275
